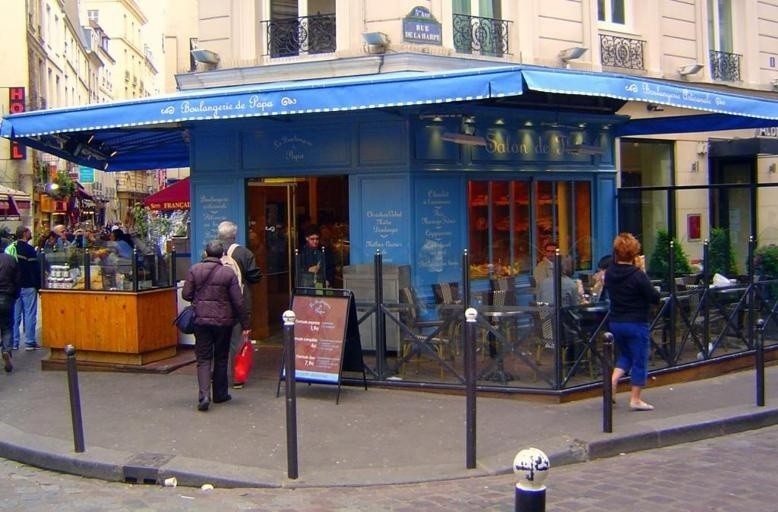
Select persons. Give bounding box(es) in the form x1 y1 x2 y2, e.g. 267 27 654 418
536 254 589 373
295 224 334 296
533 243 556 298
0 237 21 372
210 221 264 389
13 225 41 350
604 233 659 410
588 254 614 365
44 222 134 259
182 240 252 410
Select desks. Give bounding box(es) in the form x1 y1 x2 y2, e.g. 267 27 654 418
457 311 526 385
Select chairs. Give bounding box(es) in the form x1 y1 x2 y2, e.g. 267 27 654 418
481 289 518 362
430 281 464 360
398 284 455 380
527 273 778 380
490 274 519 342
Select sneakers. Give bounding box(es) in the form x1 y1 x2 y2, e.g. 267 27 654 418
233 384 244 389
214 395 231 402
25 344 41 350
199 398 209 410
2 351 13 371
631 401 654 410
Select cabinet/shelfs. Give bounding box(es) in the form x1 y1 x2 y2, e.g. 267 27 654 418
342 264 411 357
37 240 184 366
50 211 94 232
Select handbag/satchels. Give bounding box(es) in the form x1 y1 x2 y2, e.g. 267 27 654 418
234 341 253 382
172 308 195 334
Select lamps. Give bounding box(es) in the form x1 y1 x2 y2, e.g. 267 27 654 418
191 50 221 65
361 30 391 47
72 133 118 171
679 64 704 75
425 114 614 132
560 46 588 59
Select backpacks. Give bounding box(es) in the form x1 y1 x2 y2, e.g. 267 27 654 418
220 244 244 295
